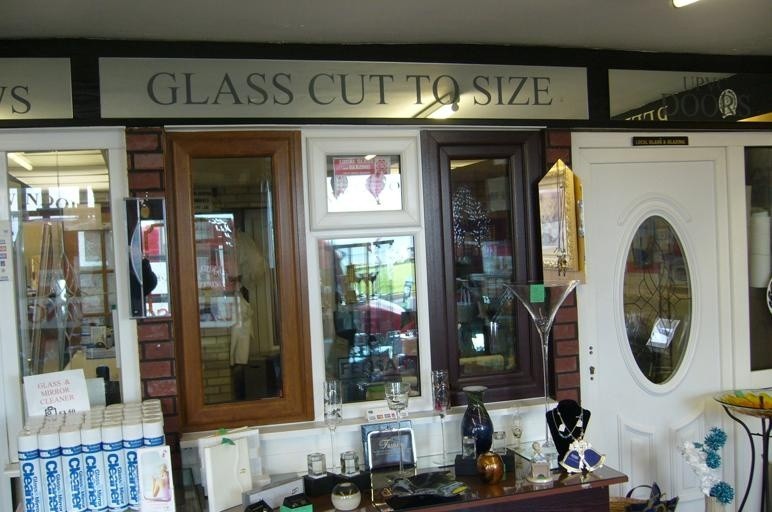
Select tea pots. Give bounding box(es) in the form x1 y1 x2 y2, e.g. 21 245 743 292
537 155 587 289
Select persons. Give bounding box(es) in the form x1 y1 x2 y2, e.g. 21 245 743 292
543 399 593 458
531 440 544 462
150 463 171 499
230 283 256 402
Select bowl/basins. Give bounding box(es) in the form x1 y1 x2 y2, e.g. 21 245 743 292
550 405 585 444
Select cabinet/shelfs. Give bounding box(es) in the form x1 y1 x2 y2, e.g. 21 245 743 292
18 399 168 512
474 452 505 485
331 482 362 511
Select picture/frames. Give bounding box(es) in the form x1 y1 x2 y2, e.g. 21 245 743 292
308 449 631 511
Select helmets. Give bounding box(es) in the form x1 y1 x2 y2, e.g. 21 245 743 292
712 388 771 417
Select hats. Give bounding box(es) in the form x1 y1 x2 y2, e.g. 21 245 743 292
339 289 416 337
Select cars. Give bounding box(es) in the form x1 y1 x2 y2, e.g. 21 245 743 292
307 452 327 479
491 431 507 455
462 436 477 461
340 450 361 477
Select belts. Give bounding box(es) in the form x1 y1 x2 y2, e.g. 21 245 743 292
501 279 570 448
322 380 344 476
457 271 513 356
510 426 525 449
384 382 412 478
431 368 454 464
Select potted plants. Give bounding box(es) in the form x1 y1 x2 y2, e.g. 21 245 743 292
538 167 579 272
308 135 423 228
